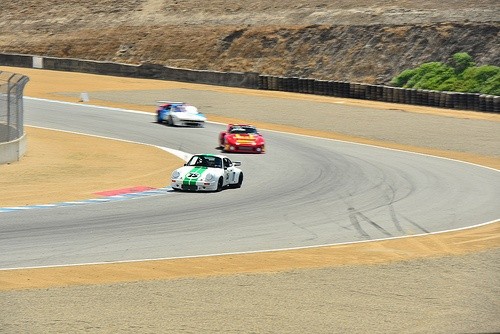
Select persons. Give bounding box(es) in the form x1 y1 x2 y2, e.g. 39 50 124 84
212 158 221 168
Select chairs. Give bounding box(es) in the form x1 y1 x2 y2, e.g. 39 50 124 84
203 159 209 165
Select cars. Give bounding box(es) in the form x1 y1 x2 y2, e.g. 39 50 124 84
171 154 246 191
221 122 265 154
156 103 207 128
